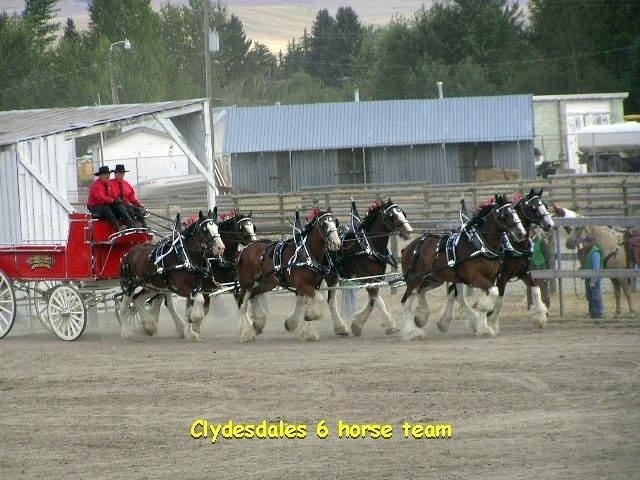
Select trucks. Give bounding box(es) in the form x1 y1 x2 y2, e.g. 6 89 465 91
575 120 640 173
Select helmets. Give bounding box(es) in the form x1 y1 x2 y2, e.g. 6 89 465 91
584 233 595 242
529 224 538 230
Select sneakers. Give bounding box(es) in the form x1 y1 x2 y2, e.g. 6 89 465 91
113 222 149 233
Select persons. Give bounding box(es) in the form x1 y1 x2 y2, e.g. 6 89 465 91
86 166 133 230
106 164 148 231
528 227 555 317
577 235 604 322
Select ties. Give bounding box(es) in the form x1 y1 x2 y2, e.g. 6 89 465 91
104 183 110 196
118 181 125 199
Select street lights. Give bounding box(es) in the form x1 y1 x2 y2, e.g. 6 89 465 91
107 37 131 104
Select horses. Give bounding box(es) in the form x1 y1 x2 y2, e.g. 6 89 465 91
565 214 640 320
435 186 555 336
302 196 413 342
237 206 349 345
118 205 256 343
400 192 528 340
551 201 579 235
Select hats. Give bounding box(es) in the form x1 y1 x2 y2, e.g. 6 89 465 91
111 164 130 173
93 165 115 176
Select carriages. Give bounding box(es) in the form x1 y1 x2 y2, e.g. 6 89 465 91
0 182 561 347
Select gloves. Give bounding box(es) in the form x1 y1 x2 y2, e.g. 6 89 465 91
113 199 123 205
589 282 596 288
